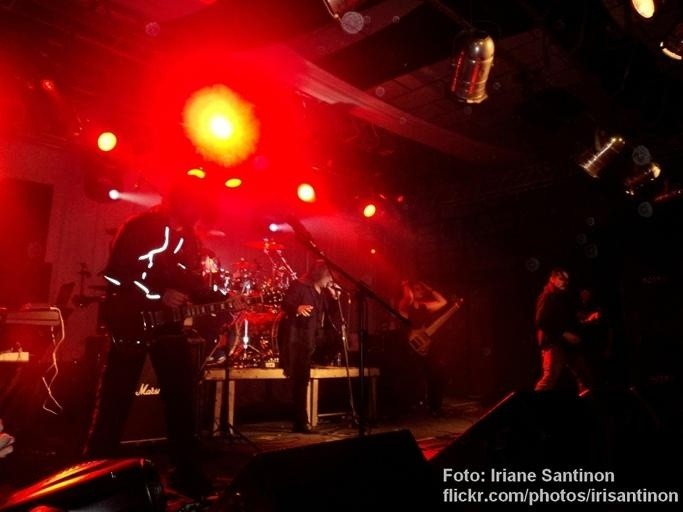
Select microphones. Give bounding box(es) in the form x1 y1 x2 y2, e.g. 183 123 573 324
328 282 350 296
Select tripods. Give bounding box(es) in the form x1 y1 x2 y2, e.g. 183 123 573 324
211 329 263 452
235 319 263 359
322 290 366 435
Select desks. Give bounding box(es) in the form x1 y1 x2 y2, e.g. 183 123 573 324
204 365 381 438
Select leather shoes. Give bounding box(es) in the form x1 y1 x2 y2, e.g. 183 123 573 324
292 422 319 434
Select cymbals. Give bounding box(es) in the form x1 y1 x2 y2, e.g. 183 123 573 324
245 241 287 251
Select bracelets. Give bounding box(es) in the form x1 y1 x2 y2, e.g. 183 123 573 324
428 287 434 295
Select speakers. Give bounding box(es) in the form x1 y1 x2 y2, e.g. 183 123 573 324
207 427 428 512
0 455 168 512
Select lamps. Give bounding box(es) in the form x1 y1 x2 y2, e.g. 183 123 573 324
660 20 683 64
450 29 495 105
630 0 666 23
577 129 627 181
623 162 663 195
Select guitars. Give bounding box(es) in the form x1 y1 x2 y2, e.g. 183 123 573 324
95 285 286 358
404 295 466 358
551 309 600 351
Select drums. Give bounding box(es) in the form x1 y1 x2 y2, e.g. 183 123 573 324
228 259 259 292
258 308 285 369
189 307 250 367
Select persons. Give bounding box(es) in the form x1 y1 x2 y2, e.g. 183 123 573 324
573 287 602 325
394 276 452 423
80 175 223 490
271 256 346 435
530 266 595 398
